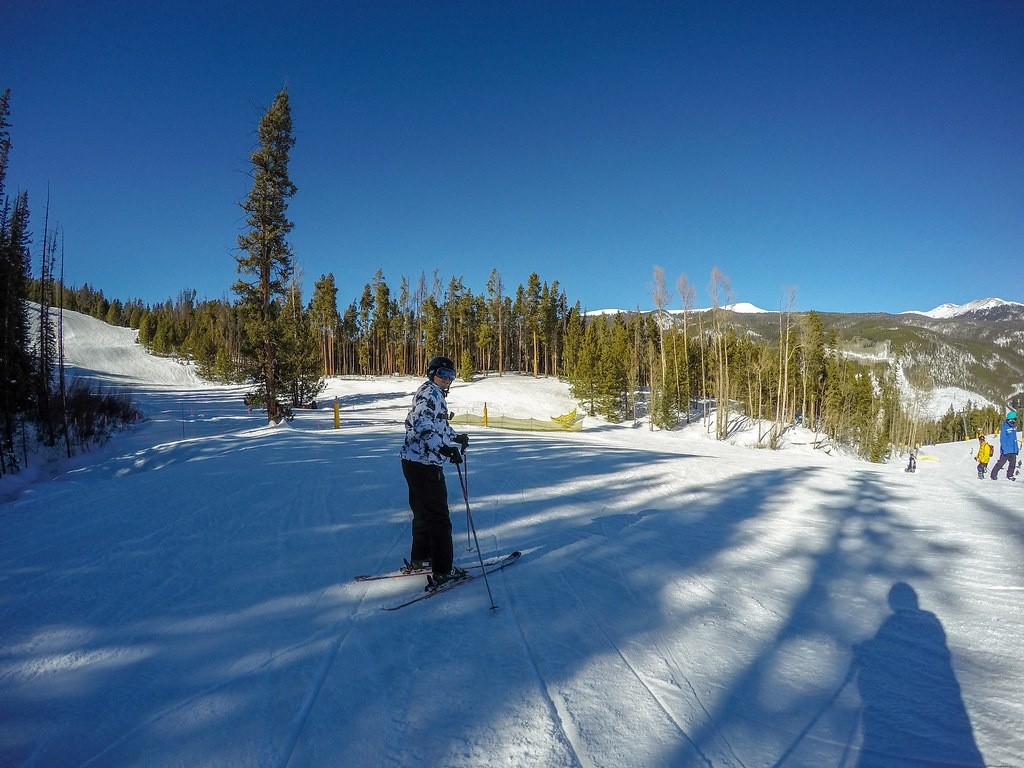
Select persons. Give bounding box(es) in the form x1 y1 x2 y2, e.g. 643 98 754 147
974 436 991 479
398 357 472 594
990 412 1019 481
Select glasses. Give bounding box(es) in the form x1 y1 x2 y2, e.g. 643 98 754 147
436 366 456 381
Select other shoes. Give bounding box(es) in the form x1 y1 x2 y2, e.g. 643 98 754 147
411 559 432 569
432 566 464 584
991 476 997 480
1008 476 1014 480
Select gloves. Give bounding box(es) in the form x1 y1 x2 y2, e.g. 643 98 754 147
440 446 463 464
454 434 469 446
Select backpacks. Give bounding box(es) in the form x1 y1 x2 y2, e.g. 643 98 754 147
987 443 994 457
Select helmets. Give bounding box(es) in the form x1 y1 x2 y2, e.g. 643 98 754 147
1006 412 1017 419
979 436 985 441
427 357 454 376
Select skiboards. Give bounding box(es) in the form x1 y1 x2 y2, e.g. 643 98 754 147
354 549 522 611
